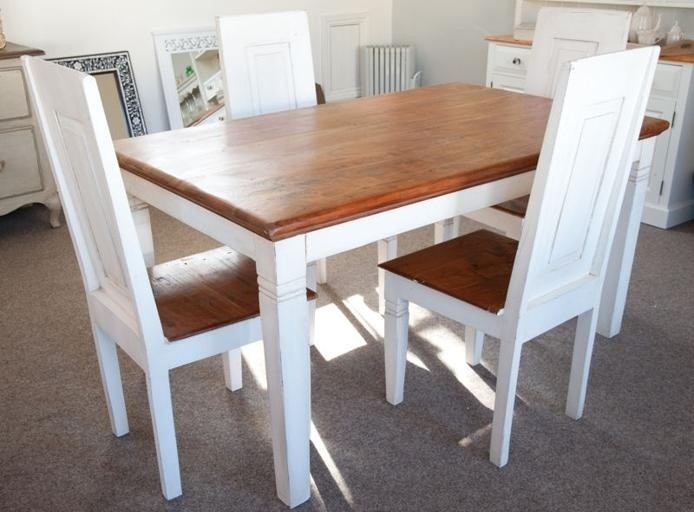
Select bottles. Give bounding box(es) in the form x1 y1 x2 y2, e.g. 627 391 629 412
184 64 194 78
180 95 206 123
628 3 685 46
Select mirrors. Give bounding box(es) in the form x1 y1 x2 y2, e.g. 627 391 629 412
45 50 148 141
152 26 226 131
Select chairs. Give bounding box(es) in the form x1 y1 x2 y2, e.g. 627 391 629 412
372 40 667 475
445 5 635 256
215 8 401 316
17 56 319 511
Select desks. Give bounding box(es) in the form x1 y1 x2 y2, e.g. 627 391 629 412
104 77 673 510
174 72 197 107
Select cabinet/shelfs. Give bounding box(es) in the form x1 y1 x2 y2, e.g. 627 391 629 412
483 35 693 230
0 41 62 230
191 44 226 128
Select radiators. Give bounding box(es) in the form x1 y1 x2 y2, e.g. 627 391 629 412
355 42 422 98
